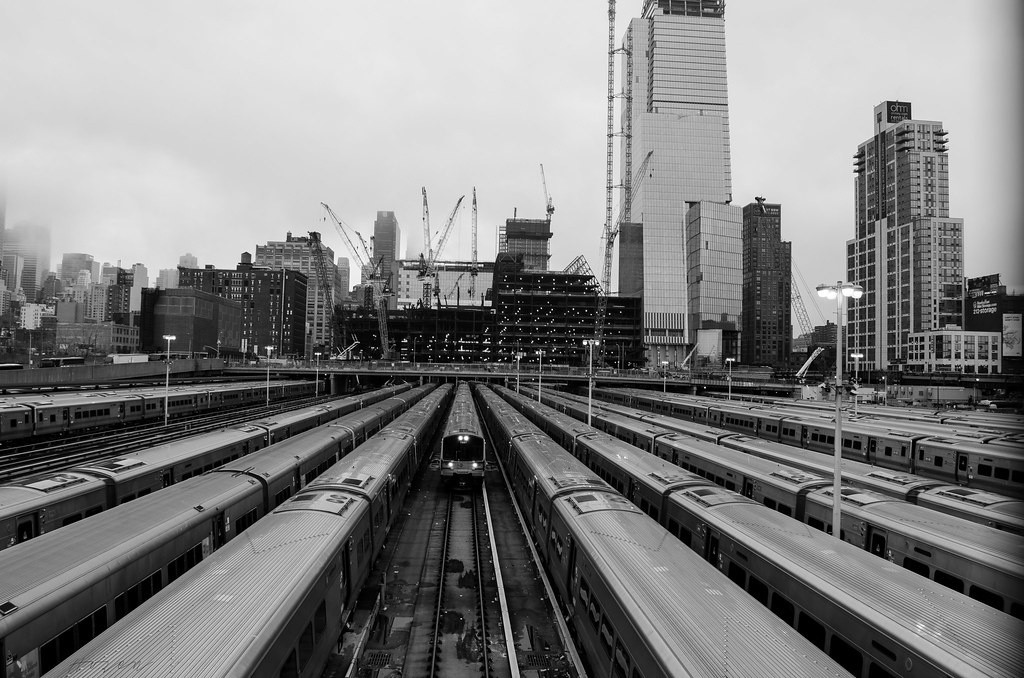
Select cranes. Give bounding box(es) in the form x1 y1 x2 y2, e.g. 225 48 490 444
587 147 653 367
538 163 555 222
319 184 479 362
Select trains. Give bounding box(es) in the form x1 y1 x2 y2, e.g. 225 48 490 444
36 380 455 678
438 381 488 494
0 379 440 678
490 382 1024 678
474 381 855 678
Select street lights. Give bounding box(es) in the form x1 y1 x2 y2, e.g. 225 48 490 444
162 334 177 427
662 361 669 392
815 280 865 540
726 357 736 400
314 352 322 397
535 349 546 403
514 355 522 394
583 338 600 426
264 346 274 407
850 353 864 419
